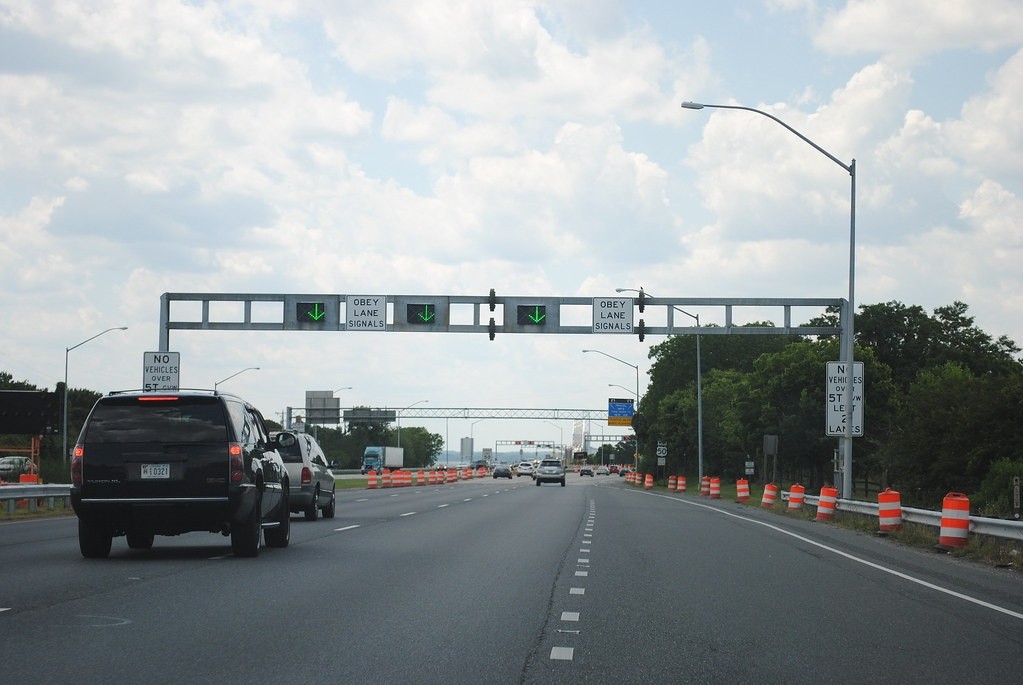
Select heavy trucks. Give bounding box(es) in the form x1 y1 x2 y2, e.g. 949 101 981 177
360 446 404 475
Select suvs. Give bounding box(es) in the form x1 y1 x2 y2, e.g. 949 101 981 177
269 432 340 520
535 458 568 487
516 462 535 477
71 387 295 558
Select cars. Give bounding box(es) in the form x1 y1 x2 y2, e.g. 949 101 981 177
493 464 513 479
471 460 491 469
0 456 35 478
491 461 521 470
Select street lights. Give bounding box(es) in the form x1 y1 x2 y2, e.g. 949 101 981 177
63 327 128 469
398 400 428 447
616 288 702 488
583 349 638 472
543 421 562 457
215 368 260 390
589 421 603 465
681 101 856 498
